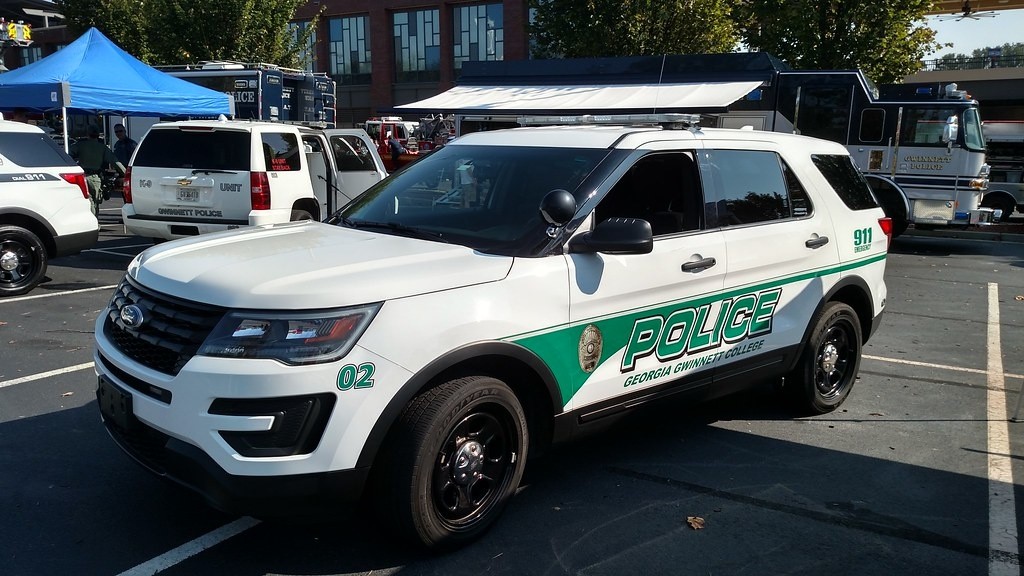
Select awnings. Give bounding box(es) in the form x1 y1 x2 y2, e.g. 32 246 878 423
391 78 763 113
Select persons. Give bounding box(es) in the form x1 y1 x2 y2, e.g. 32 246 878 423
388 135 406 171
114 123 139 224
68 125 127 219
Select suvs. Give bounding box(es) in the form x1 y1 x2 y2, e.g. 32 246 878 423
122 115 400 242
96 122 891 558
1 119 101 297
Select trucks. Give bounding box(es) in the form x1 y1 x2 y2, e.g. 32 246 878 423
976 120 1024 215
394 50 992 248
99 60 337 174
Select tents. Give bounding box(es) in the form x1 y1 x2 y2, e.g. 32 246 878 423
0 26 235 154
